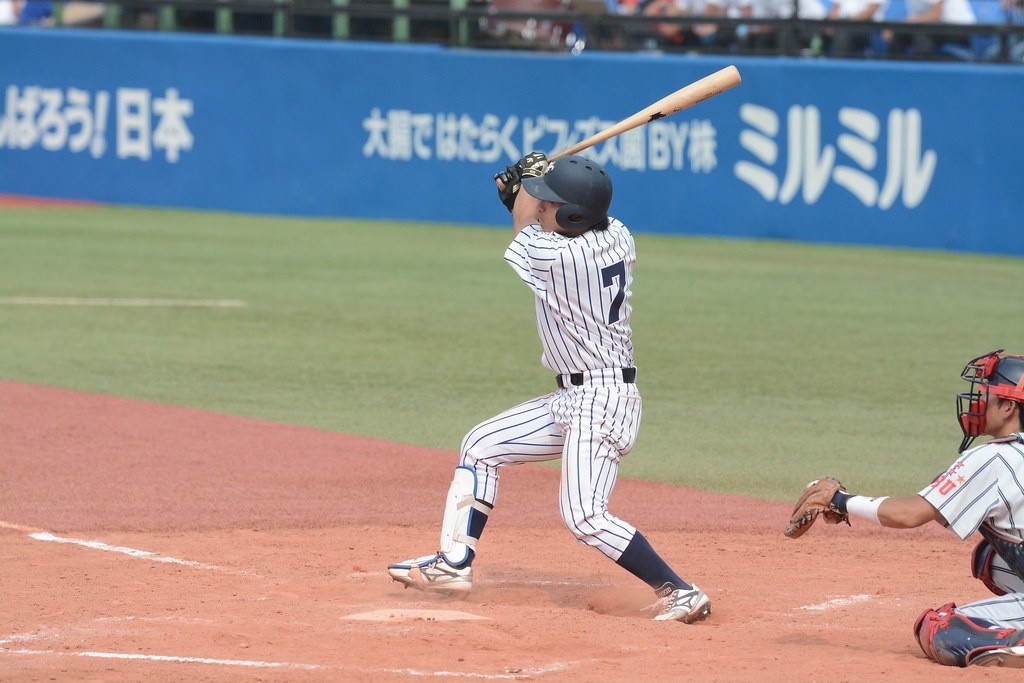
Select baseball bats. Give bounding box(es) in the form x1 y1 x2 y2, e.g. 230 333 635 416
495 63 743 191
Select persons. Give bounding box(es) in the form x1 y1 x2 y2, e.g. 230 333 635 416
485 0 1024 61
387 151 713 624
785 349 1024 668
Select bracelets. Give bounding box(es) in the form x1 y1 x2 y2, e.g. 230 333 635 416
846 495 888 527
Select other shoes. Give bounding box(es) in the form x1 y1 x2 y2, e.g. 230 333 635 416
971 645 1024 669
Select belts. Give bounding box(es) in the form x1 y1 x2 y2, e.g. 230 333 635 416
555 367 635 389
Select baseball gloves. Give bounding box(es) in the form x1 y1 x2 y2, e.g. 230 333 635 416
782 475 846 540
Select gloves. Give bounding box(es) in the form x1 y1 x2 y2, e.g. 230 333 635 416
493 152 549 213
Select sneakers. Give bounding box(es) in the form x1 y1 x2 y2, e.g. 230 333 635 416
640 583 711 622
389 556 474 594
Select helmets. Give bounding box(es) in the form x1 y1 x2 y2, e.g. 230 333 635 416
520 156 613 231
978 356 1024 402
